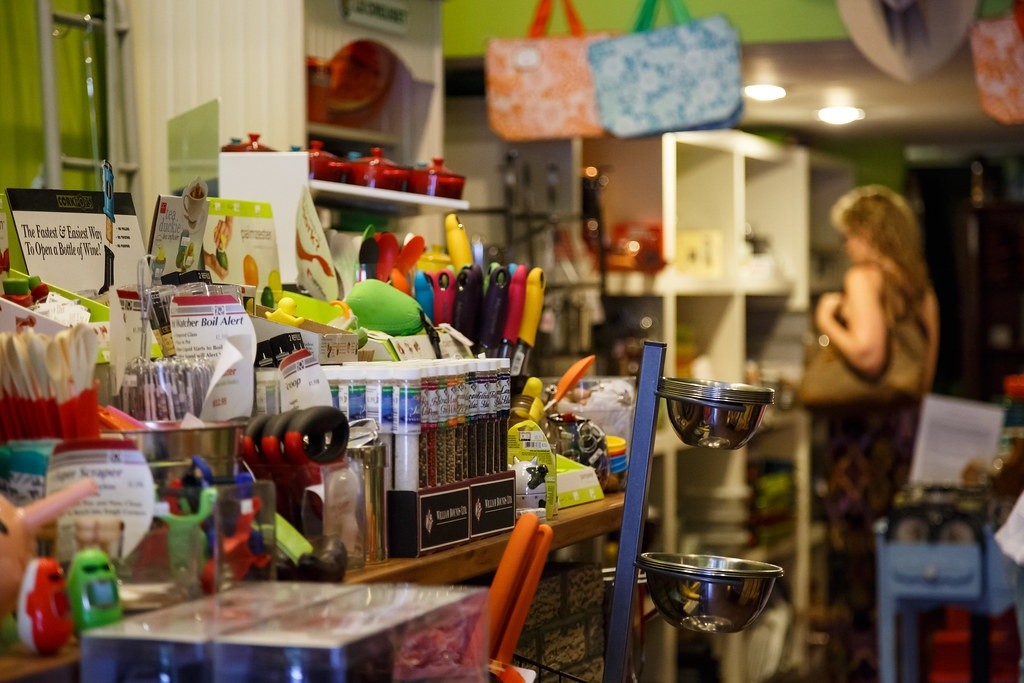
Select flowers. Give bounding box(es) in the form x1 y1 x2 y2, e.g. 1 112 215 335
1000 375 1024 413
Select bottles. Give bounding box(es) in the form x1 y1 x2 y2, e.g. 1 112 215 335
507 394 558 520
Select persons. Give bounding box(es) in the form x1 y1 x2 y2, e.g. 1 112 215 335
813 187 940 682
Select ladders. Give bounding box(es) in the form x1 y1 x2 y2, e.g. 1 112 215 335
36 1 148 248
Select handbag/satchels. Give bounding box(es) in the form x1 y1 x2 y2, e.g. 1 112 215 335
588 1 745 139
796 258 931 406
968 0 1024 125
485 0 611 142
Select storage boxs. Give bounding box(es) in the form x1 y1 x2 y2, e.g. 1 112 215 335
384 468 519 560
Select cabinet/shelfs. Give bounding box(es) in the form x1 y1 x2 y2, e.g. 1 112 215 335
596 129 864 683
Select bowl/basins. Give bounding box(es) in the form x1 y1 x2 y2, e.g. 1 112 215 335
605 435 628 493
659 376 775 450
222 133 466 199
638 552 785 635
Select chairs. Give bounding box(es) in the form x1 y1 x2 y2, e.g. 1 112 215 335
869 486 1011 683
